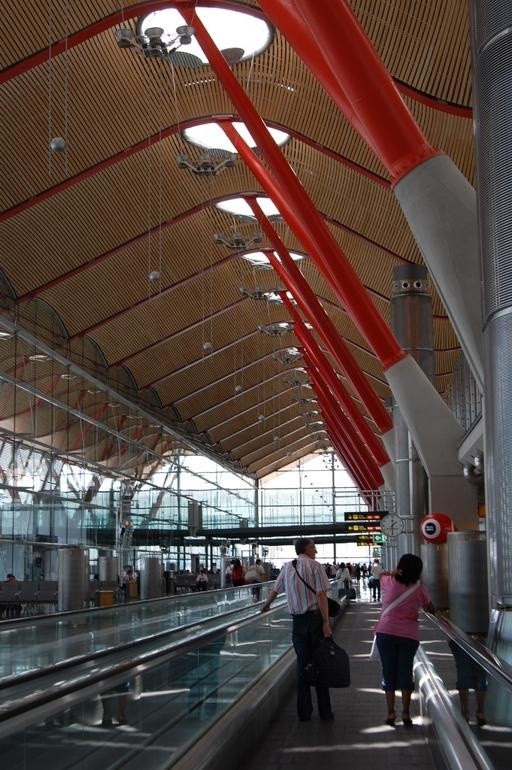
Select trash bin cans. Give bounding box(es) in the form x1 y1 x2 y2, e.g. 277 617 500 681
95 591 114 607
128 581 138 598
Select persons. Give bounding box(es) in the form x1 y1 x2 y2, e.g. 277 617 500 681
223 557 384 600
192 570 210 589
100 680 131 726
445 638 488 722
260 535 354 721
182 624 226 725
5 573 23 612
374 551 431 727
122 569 134 601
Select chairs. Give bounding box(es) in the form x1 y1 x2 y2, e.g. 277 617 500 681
175 573 221 593
0 580 97 618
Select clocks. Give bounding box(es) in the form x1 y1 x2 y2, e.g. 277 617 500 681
380 513 404 538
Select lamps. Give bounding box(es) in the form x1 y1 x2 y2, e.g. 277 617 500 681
116 0 329 456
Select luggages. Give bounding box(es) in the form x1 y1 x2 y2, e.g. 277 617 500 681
347 582 356 599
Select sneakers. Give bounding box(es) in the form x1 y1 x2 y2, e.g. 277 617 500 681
321 712 334 720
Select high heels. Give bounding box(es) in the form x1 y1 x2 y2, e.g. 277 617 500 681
403 719 412 726
385 719 396 726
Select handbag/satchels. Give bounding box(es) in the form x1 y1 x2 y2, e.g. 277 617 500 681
244 566 260 583
327 598 340 617
300 635 351 690
370 634 381 659
